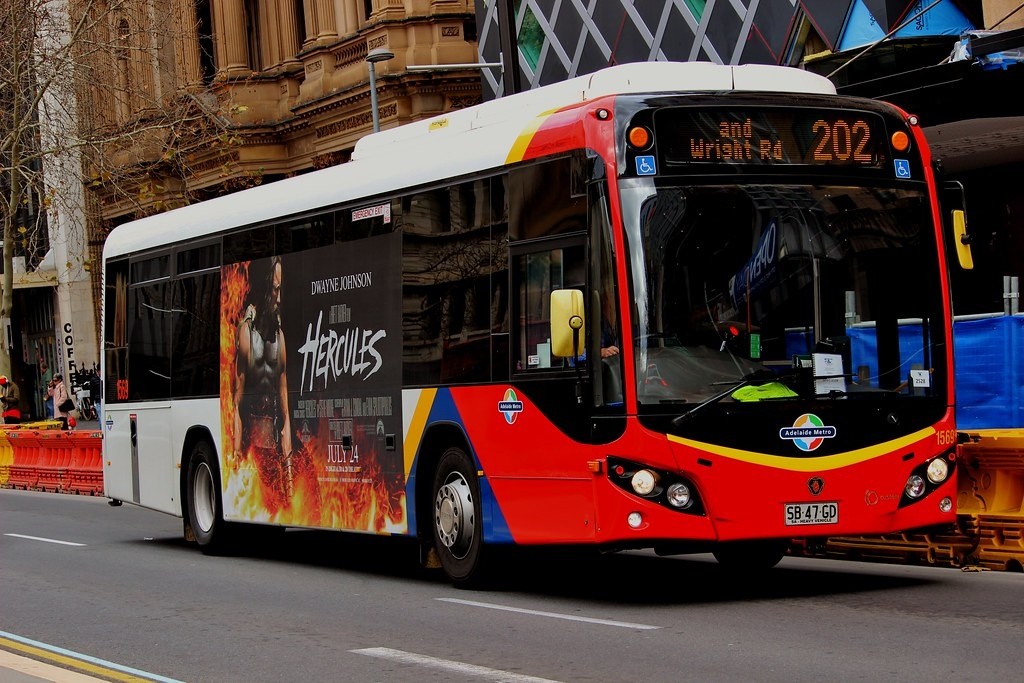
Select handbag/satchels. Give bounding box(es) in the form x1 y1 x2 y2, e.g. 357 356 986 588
59 387 75 413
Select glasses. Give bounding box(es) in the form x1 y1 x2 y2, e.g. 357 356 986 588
53 380 58 382
273 284 280 293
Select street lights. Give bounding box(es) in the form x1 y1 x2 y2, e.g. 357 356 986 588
363 47 395 133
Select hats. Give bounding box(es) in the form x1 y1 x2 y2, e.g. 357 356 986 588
0 375 9 384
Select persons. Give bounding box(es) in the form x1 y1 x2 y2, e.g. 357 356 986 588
90 363 102 430
38 362 54 420
108 498 122 507
232 255 294 503
47 373 69 436
0 375 35 424
563 271 654 372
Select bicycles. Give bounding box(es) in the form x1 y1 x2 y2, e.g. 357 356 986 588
74 380 99 421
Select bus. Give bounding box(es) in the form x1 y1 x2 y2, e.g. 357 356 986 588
98 62 974 591
